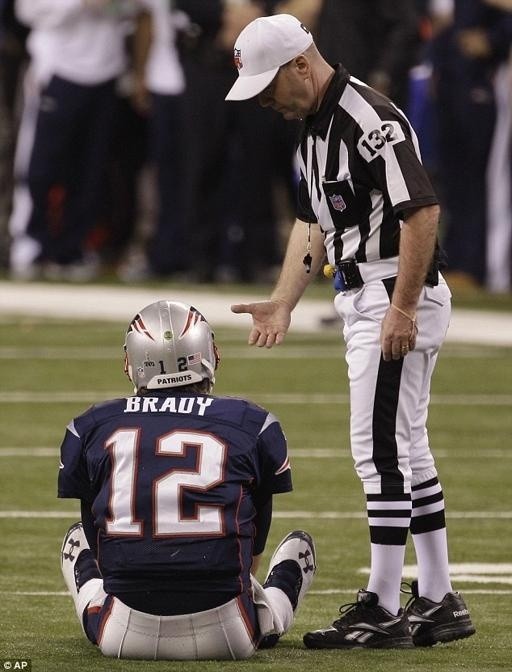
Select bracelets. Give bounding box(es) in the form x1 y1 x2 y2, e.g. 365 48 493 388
390 302 418 322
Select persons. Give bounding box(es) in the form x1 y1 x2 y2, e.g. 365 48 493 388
57 295 316 658
0 1 510 297
224 9 478 651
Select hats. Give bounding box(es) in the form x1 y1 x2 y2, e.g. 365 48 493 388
224 13 314 102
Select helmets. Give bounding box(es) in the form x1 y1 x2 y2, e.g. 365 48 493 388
123 299 220 392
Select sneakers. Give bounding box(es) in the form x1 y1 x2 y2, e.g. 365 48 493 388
59 520 103 601
303 578 476 651
261 530 316 621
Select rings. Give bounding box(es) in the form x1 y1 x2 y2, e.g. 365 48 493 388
402 346 408 350
